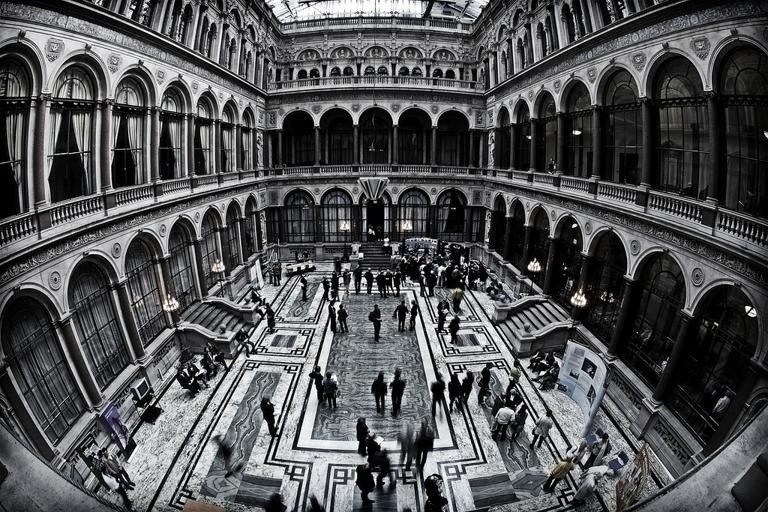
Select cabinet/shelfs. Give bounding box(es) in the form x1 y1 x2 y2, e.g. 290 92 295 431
132 387 165 425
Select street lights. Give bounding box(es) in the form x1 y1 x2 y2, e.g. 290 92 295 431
212 258 227 299
340 221 350 263
561 284 588 359
526 256 542 295
162 292 186 351
596 289 615 327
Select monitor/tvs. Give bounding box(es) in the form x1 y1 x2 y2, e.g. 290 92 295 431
130 376 150 401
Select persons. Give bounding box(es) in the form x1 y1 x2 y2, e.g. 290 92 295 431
246 237 488 344
702 389 732 432
97 447 136 511
212 346 631 511
177 340 228 396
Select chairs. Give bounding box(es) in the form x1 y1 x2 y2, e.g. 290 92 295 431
608 451 630 474
